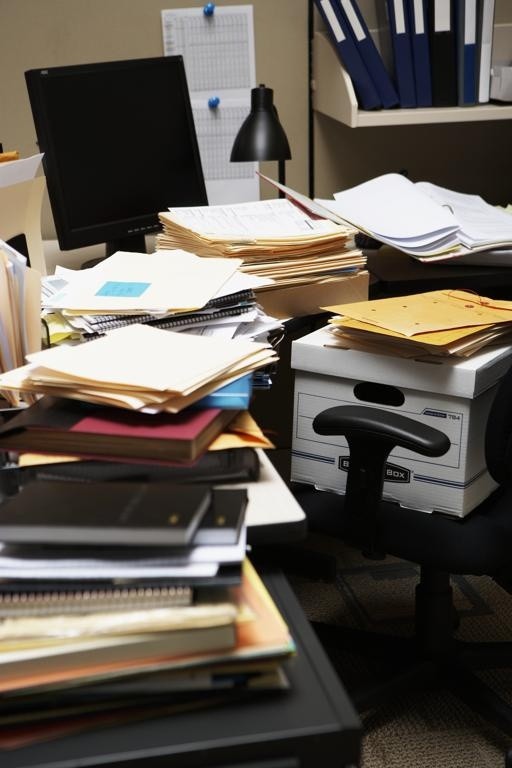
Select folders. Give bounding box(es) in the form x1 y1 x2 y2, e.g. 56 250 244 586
313 0 498 110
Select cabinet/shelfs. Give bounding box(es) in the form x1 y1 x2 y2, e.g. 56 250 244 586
307 4 512 293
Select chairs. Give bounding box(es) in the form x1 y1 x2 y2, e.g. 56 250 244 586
288 361 511 732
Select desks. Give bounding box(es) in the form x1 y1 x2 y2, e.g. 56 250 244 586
0 269 378 768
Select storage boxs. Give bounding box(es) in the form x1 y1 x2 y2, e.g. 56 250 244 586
291 319 511 517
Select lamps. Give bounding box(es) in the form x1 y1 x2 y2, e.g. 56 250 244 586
228 78 294 198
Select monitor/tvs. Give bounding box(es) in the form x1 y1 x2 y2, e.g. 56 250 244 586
24 55 209 270
